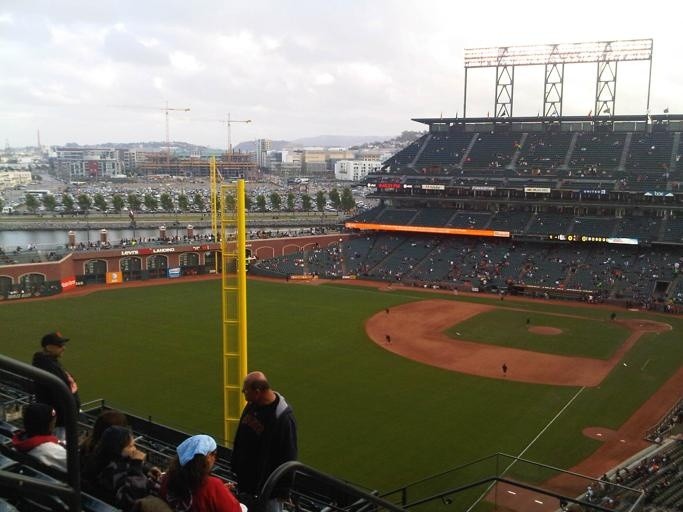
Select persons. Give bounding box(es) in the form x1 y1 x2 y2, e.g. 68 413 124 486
502 363 508 377
0 225 237 298
31 331 81 444
9 402 67 472
609 311 615 323
488 128 682 189
103 425 168 512
385 334 392 345
557 404 683 512
243 191 682 319
231 370 299 512
160 433 251 512
78 407 129 512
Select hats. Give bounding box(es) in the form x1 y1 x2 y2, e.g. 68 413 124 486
41 332 69 347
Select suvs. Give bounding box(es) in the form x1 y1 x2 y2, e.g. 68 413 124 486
155 101 189 163
216 110 251 163
